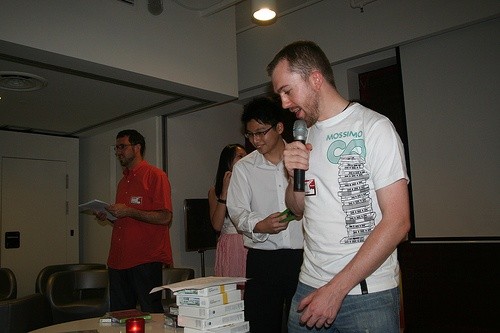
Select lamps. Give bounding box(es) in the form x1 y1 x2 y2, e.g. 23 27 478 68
251 0 276 21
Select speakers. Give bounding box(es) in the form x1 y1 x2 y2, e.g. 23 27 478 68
184 199 218 252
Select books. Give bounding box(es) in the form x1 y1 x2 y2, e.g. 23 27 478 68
78 199 118 221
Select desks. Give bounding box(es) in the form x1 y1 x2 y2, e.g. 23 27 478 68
29 312 182 333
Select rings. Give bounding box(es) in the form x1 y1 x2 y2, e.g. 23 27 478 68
326 321 331 325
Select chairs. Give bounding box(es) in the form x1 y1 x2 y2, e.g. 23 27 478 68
0 263 195 333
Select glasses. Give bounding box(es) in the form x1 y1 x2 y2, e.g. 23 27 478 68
113 143 136 152
245 120 278 138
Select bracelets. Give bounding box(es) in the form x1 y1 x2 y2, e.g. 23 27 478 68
217 199 226 203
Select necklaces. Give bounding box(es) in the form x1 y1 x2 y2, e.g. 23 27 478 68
342 102 350 111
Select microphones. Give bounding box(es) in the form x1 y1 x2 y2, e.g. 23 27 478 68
292 119 309 191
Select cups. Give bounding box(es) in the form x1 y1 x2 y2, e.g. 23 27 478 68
126 318 145 333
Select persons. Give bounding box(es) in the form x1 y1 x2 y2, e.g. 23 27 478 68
95 129 172 313
227 97 303 333
267 40 410 333
208 144 248 277
